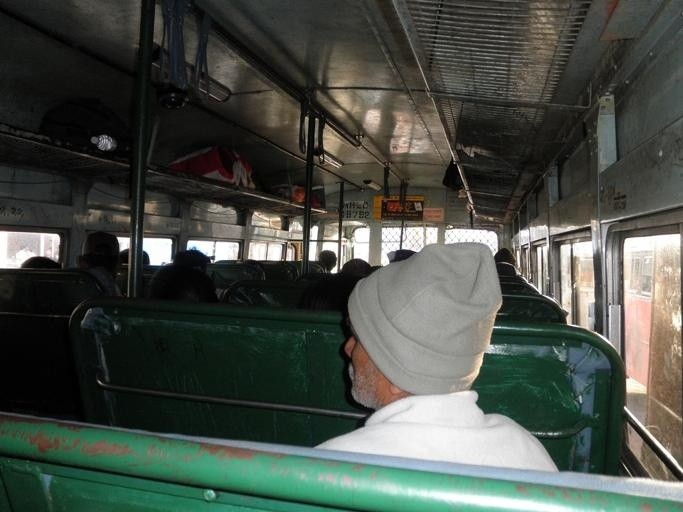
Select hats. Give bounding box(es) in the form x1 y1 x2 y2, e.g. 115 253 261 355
82 231 118 261
349 242 503 395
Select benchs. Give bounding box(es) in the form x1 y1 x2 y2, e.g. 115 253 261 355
0 259 683 512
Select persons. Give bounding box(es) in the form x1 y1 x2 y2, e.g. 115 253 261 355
314 243 560 472
